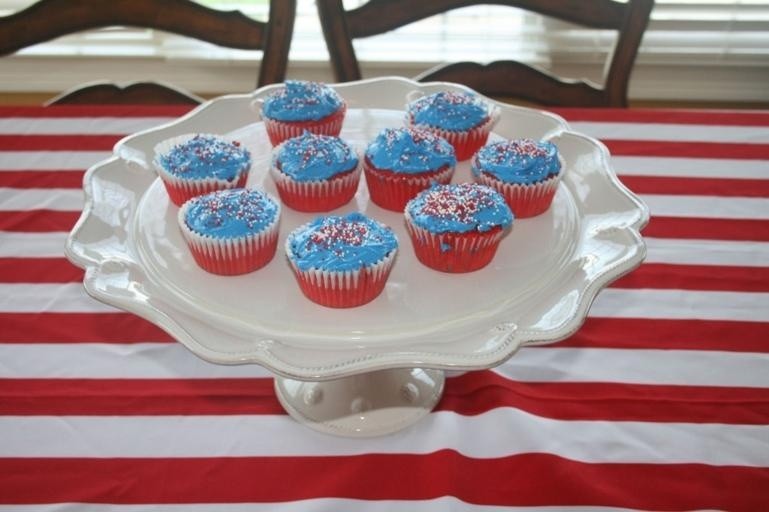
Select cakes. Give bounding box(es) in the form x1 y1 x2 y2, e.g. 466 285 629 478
403 182 514 274
407 90 501 162
362 124 457 213
151 132 252 206
269 128 362 212
177 186 281 276
284 212 398 308
472 138 567 220
263 80 347 147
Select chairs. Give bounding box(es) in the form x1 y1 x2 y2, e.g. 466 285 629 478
315 0 655 108
0 0 297 106
